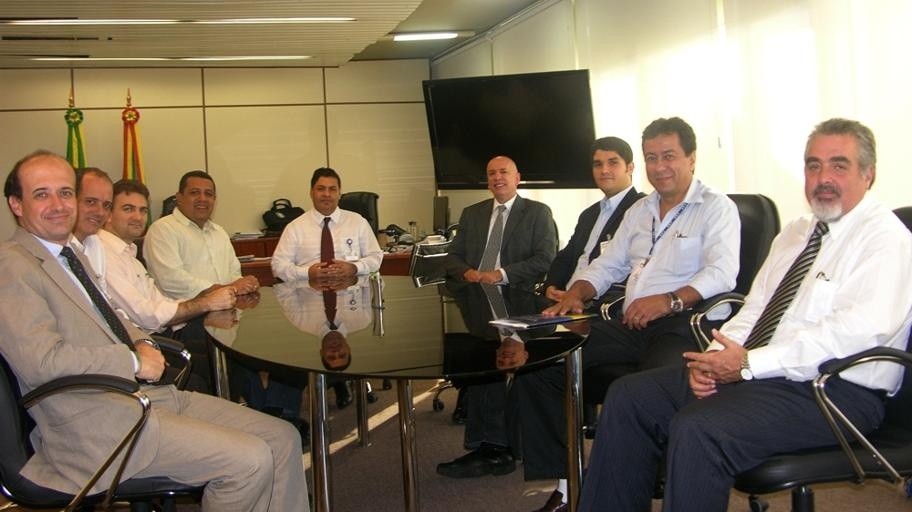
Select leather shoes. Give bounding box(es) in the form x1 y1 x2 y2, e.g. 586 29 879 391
434 444 518 480
333 379 354 410
530 488 569 512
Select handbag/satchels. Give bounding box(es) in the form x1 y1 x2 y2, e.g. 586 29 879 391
261 197 306 232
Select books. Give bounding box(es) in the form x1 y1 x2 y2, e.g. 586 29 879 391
230 228 264 242
237 254 256 263
488 311 599 330
254 256 271 261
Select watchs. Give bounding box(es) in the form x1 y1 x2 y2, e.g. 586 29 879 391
738 350 755 382
667 292 685 315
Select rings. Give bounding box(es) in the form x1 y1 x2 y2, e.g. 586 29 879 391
632 316 640 321
708 373 713 379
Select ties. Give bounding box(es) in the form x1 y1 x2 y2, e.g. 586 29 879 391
58 245 138 353
321 289 338 331
741 219 830 352
480 281 515 338
477 205 507 273
320 217 336 269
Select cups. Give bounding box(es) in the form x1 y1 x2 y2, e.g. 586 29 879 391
368 277 383 309
370 309 385 338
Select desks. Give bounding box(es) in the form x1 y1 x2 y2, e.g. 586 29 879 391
239 252 414 287
202 273 584 512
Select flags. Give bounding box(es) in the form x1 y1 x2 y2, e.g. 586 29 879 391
120 105 153 239
59 88 88 169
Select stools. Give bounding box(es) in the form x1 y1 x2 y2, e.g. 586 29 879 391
355 372 396 402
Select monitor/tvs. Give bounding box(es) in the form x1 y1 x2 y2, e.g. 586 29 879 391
434 197 448 234
445 332 584 373
422 69 596 190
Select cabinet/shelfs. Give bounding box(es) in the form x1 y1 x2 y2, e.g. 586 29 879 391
231 236 280 257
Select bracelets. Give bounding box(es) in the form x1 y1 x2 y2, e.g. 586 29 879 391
140 338 159 349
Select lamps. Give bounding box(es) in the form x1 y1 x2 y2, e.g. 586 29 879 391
378 29 477 42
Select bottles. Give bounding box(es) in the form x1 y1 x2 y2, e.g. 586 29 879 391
407 220 419 242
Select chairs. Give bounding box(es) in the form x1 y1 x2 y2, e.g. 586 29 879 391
0 333 213 510
600 192 782 403
689 203 912 512
407 238 451 280
430 216 561 421
338 191 380 241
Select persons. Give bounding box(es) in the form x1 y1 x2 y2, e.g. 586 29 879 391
204 308 241 331
272 165 384 410
269 273 385 372
518 116 742 512
72 166 114 299
570 116 912 512
436 135 651 481
451 282 560 371
94 177 239 396
139 169 311 441
0 149 314 512
443 153 559 425
207 291 262 346
